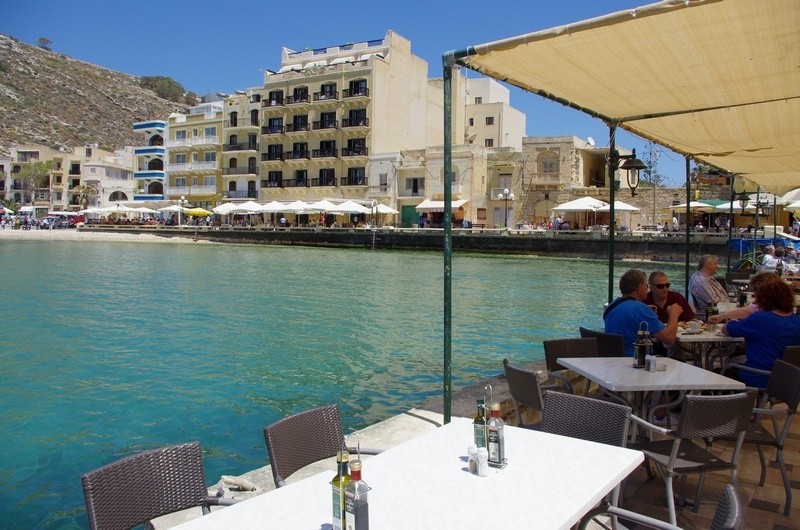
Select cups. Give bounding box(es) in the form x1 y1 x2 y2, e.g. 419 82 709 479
690 321 701 330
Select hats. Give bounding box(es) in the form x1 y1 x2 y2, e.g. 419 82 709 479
786 242 794 249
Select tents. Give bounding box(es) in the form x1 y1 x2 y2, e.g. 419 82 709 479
442 0 800 424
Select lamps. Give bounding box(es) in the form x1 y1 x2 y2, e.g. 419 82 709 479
731 189 751 214
612 148 649 198
757 200 772 220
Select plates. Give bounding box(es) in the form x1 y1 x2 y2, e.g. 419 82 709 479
686 328 703 333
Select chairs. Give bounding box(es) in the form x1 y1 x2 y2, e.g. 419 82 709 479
503 278 800 530
544 222 629 236
661 226 755 238
264 403 384 489
82 440 240 530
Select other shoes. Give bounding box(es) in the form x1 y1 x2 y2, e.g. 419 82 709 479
653 414 681 425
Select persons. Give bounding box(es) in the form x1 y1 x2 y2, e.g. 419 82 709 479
689 254 729 309
0 215 52 231
636 224 641 231
603 269 682 357
762 241 800 266
696 221 703 231
419 212 428 228
709 272 800 389
308 214 326 227
643 272 695 324
792 217 800 235
206 214 210 226
100 212 172 226
225 215 230 224
543 216 579 230
715 215 721 233
657 214 677 232
280 216 292 227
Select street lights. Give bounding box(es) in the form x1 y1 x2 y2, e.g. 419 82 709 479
178 195 188 225
497 188 515 227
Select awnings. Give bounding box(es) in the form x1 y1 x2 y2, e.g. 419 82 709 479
416 199 468 212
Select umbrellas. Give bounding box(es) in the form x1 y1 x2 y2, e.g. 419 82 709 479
212 200 400 224
552 196 640 213
81 204 213 217
669 188 800 215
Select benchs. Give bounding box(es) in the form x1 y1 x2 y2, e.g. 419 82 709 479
515 223 537 235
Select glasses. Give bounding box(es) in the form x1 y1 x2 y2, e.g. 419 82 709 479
641 284 649 288
651 283 671 289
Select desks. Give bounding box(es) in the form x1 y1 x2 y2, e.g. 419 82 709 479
165 417 644 530
556 356 746 506
678 324 744 370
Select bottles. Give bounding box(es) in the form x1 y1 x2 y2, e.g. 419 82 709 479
706 300 713 321
477 447 488 476
467 443 478 473
713 300 719 315
737 287 747 306
332 450 351 530
473 400 487 448
345 459 369 530
633 330 656 371
776 260 783 277
487 403 504 467
